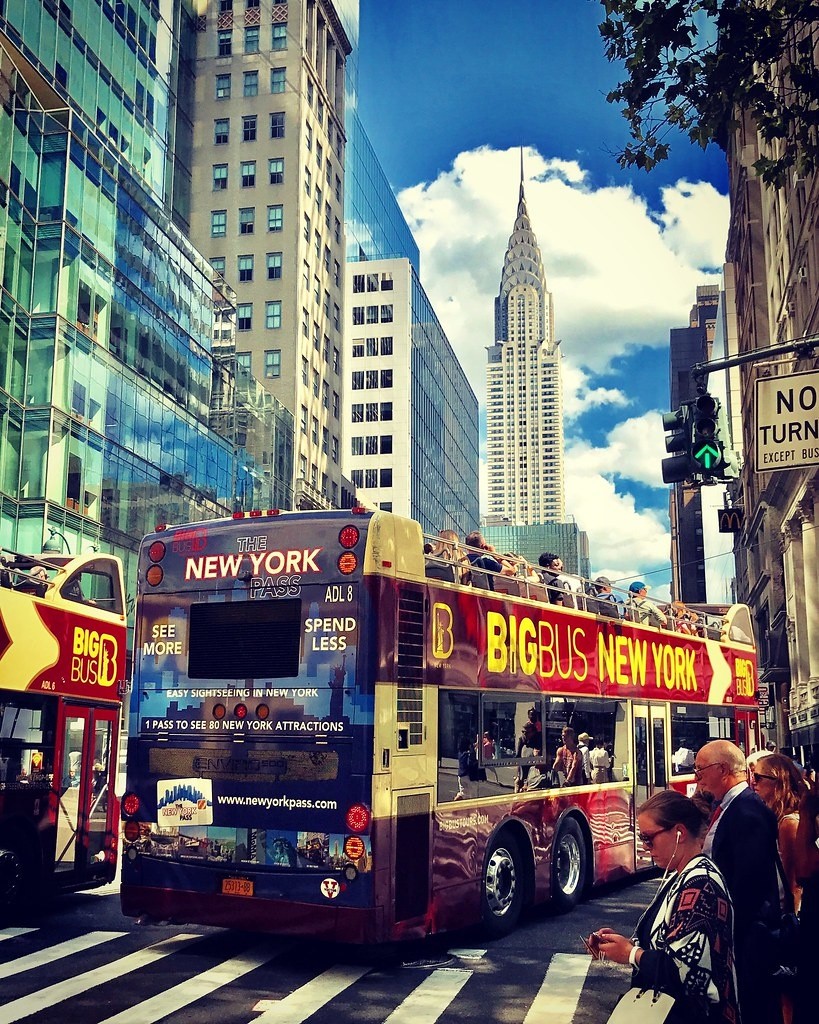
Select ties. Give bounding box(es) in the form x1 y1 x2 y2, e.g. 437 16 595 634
701 805 722 849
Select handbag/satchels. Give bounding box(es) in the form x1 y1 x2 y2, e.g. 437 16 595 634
753 908 798 946
605 987 678 1024
469 761 487 781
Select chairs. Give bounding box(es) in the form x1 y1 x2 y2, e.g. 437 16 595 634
576 586 600 615
618 599 640 623
650 608 674 630
599 594 620 619
425 547 456 583
471 557 491 590
519 572 550 603
563 579 578 609
493 572 522 597
707 621 722 642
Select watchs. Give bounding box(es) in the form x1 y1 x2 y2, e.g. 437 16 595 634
629 946 642 972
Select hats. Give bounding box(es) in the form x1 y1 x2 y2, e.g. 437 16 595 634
578 732 593 740
767 741 776 747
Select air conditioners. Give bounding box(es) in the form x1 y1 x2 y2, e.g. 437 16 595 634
787 302 795 318
797 267 808 284
792 171 807 189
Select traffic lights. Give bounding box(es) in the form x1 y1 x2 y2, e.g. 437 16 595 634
661 406 694 484
690 396 724 477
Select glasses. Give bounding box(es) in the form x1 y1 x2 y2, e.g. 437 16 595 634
638 828 673 848
754 772 777 783
522 730 530 734
694 762 722 779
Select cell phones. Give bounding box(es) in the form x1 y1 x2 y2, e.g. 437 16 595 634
592 932 608 944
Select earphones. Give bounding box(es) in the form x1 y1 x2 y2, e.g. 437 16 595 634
676 830 681 844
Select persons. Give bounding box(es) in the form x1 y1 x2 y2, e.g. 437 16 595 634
585 789 742 1024
454 708 610 800
690 740 819 1024
29 567 97 605
424 530 752 644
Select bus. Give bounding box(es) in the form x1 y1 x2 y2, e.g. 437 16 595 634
0 549 125 896
120 509 758 939
